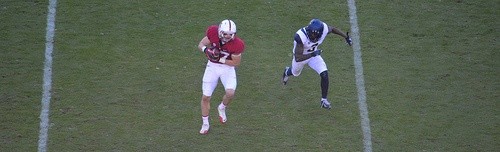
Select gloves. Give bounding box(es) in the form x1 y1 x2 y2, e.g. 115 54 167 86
345 31 352 46
204 47 223 64
311 50 323 57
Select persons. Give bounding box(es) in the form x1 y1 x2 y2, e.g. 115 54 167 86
281 17 353 110
197 19 246 136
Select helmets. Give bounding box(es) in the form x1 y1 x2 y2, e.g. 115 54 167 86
307 18 324 43
218 20 237 40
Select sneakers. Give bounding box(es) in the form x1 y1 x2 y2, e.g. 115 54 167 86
281 67 290 86
200 123 209 134
320 97 330 109
218 105 227 123
